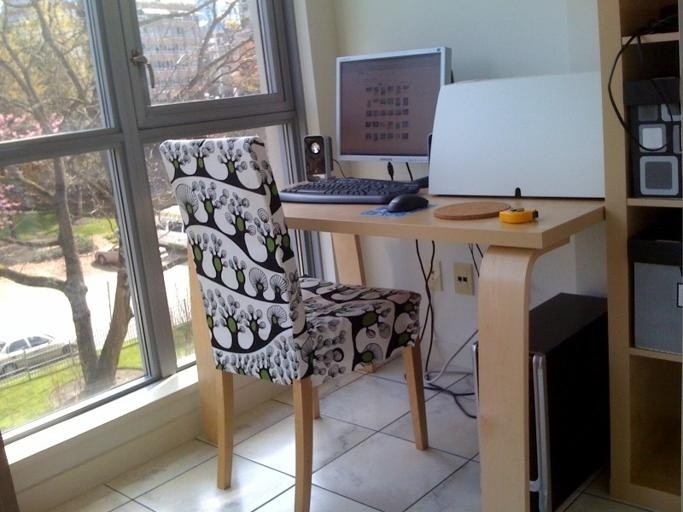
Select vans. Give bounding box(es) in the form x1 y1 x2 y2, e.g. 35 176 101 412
156 205 189 253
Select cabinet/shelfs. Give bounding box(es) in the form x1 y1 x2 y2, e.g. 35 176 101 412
596 0 683 512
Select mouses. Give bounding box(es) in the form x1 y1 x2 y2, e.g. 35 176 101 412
386 193 430 213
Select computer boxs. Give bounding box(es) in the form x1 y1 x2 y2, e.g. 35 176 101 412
472 292 610 511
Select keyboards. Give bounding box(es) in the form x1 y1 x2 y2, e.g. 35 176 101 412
279 178 419 203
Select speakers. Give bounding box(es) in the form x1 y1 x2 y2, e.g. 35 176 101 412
303 135 333 182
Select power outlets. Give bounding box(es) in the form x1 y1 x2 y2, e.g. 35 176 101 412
424 259 443 293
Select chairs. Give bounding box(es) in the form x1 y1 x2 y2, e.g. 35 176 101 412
159 133 430 512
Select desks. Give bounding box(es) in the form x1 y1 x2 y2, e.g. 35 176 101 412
280 192 606 512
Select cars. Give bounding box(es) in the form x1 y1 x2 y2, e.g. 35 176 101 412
95 240 169 272
0 330 77 379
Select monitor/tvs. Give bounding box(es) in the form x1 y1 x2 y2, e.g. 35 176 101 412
335 45 452 181
